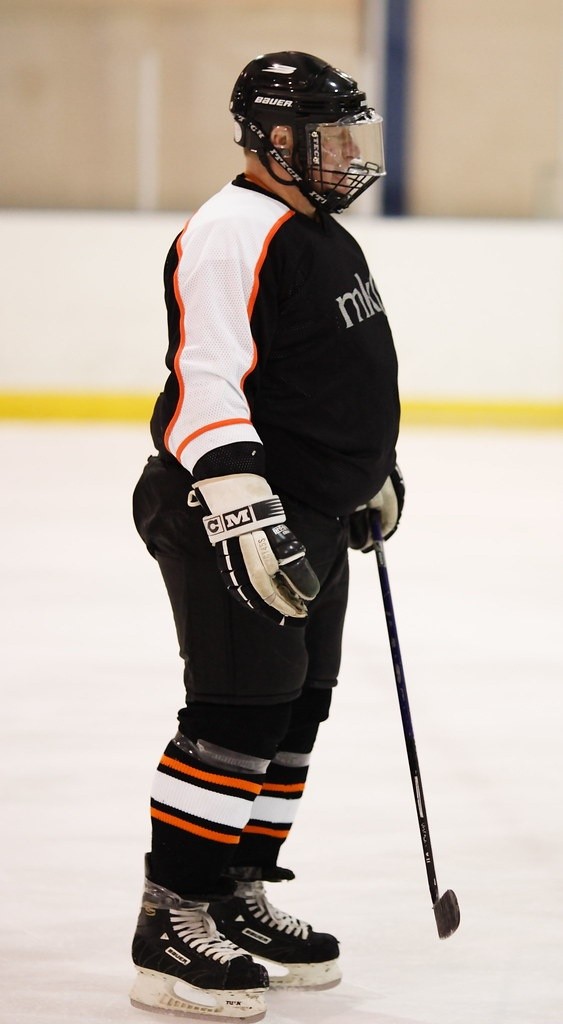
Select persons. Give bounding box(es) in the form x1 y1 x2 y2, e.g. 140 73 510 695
133 51 405 992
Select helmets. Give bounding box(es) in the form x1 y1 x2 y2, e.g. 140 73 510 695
229 50 386 215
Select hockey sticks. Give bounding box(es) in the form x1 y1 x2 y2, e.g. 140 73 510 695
368 508 466 943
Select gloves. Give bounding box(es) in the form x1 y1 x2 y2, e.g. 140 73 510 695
346 465 405 554
187 474 321 629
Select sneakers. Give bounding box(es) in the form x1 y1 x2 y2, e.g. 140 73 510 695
127 852 269 1018
209 867 341 988
